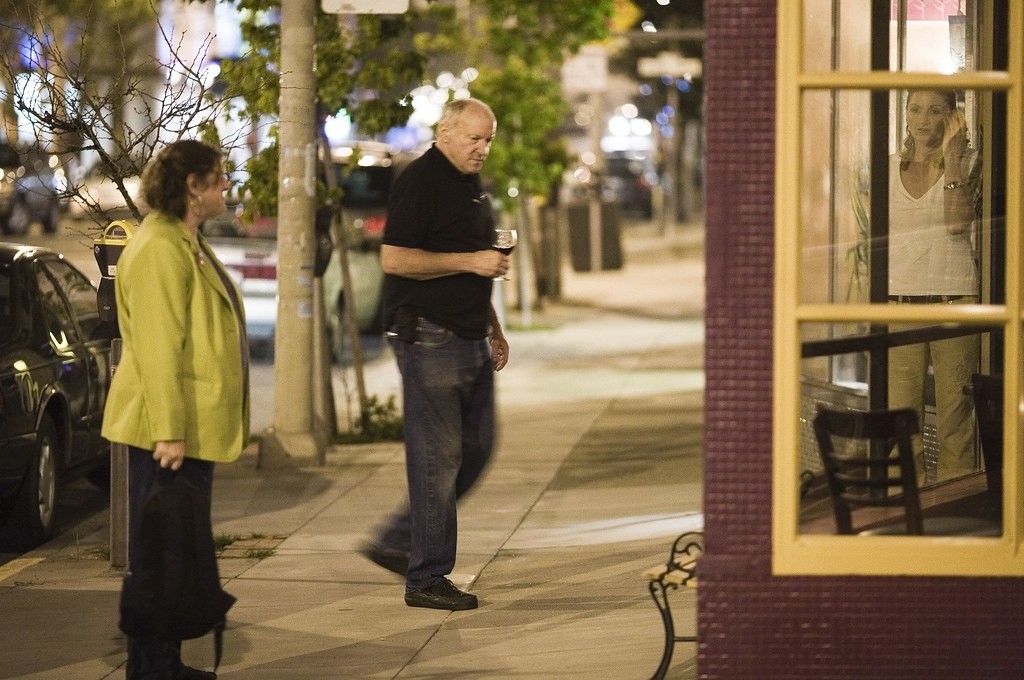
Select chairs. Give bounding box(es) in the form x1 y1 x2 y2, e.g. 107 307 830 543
812 404 1002 537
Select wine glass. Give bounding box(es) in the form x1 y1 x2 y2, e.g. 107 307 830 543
490 230 518 281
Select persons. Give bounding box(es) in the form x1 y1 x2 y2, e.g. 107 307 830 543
361 98 510 609
888 85 984 514
100 140 249 680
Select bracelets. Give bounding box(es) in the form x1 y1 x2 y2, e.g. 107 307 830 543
943 181 961 192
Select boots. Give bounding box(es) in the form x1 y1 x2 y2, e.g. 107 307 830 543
124 637 217 680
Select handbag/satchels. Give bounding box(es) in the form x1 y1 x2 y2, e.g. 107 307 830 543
119 467 236 638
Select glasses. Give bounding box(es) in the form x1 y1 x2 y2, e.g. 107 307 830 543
210 169 230 182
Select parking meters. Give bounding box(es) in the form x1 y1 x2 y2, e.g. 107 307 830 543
93 218 139 568
316 181 335 445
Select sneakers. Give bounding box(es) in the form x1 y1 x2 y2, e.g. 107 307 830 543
360 539 411 576
405 578 478 611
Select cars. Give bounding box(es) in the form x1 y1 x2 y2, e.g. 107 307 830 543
206 200 388 344
1 244 115 554
576 145 666 218
317 136 418 210
1 137 64 239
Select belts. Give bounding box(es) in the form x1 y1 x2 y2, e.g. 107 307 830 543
888 294 963 303
